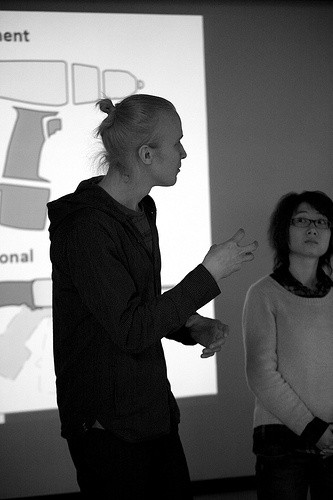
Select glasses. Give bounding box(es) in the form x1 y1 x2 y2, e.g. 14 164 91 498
288 218 332 229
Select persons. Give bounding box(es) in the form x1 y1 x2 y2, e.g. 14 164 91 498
46 94 258 500
241 188 333 499
